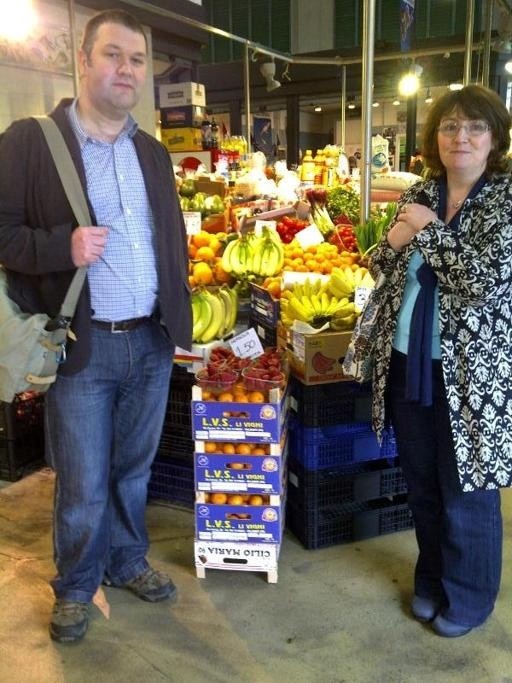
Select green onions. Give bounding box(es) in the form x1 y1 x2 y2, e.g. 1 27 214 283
311 201 335 234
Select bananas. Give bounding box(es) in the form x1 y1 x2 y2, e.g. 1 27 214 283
189 226 374 346
312 351 336 374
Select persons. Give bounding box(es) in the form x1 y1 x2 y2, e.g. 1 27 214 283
367 85 512 636
0 8 193 650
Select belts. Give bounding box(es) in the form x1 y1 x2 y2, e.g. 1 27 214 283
91 316 154 333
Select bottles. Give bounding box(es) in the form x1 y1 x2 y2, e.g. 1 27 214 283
220 136 247 155
300 149 340 187
227 156 241 186
201 120 218 148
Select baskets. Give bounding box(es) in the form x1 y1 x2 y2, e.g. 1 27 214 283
146 362 195 503
0 400 45 482
289 373 416 550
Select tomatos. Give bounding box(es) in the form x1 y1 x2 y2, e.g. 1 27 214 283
276 215 308 244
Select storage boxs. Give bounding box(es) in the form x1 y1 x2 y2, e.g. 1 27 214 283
157 81 209 151
273 317 363 386
245 277 284 349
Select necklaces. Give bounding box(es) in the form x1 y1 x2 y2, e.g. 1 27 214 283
447 197 463 209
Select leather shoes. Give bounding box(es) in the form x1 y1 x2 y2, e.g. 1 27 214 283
410 594 475 637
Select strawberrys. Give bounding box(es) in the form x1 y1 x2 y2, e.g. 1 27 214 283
197 345 284 393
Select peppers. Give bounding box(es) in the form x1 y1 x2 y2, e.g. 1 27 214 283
178 178 224 214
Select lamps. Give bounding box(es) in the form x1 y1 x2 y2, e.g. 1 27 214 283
255 50 282 93
394 55 426 98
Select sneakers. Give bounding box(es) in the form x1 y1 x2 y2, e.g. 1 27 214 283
106 555 177 601
50 595 91 642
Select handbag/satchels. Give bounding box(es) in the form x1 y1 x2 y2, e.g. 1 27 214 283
341 265 390 383
0 265 74 403
